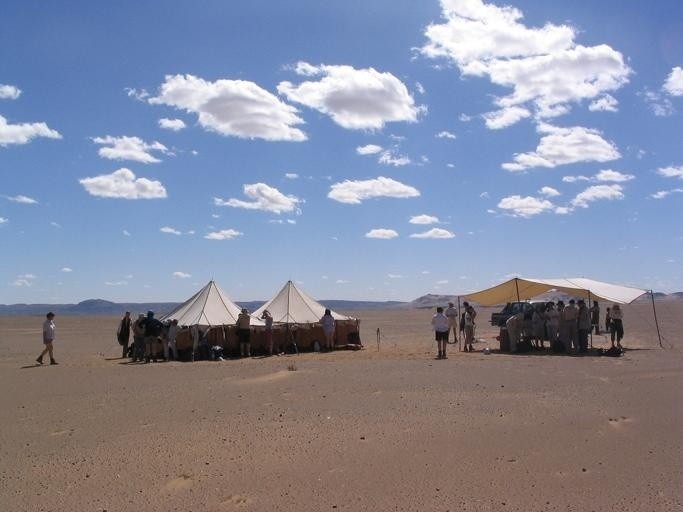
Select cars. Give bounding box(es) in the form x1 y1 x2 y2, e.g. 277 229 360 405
490 301 594 336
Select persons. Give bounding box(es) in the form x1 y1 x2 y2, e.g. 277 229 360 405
261 309 273 356
319 309 335 352
462 302 476 343
444 302 459 343
500 300 624 354
431 306 449 359
235 309 252 357
117 310 180 364
463 306 475 353
36 312 59 365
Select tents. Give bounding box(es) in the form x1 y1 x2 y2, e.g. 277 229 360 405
154 280 266 358
250 280 363 352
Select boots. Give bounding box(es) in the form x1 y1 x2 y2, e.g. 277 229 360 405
464 345 475 352
35 354 45 364
49 358 59 365
436 350 448 359
